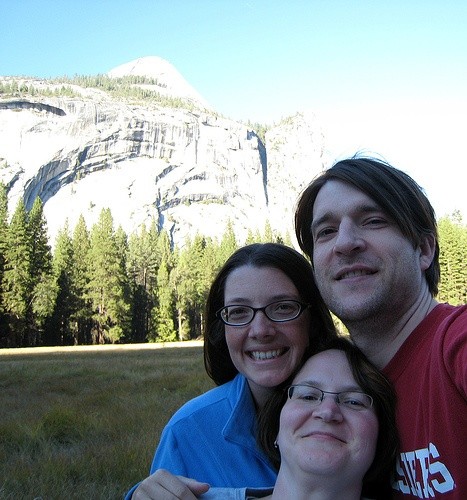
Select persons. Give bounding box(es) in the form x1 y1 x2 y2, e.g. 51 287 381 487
122 243 338 500
199 337 400 500
293 157 467 500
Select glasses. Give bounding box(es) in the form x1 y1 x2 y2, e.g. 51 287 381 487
216 299 303 326
287 384 374 411
